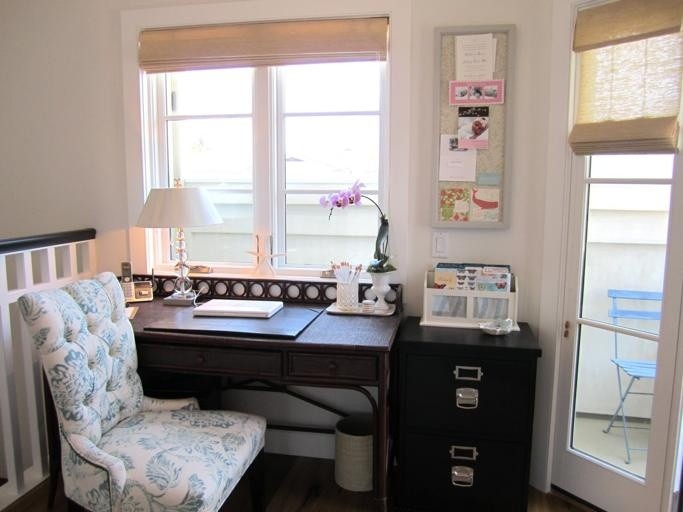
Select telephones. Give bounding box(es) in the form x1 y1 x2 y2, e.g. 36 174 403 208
121 262 153 303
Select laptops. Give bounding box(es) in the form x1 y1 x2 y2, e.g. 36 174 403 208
192 298 283 319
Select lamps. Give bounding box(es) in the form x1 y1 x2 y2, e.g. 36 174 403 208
136 178 224 305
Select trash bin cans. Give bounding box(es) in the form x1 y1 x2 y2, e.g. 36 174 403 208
333 419 377 492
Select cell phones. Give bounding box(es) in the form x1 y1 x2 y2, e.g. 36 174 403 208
120 262 131 283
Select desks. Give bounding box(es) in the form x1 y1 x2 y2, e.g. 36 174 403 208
127 296 403 511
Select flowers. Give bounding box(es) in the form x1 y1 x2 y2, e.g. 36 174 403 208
319 180 388 227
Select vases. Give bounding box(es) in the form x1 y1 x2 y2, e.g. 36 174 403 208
367 221 396 272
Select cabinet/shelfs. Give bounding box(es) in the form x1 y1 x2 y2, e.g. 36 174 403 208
395 315 543 510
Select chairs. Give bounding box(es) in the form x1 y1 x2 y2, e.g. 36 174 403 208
20 269 267 512
604 288 663 464
